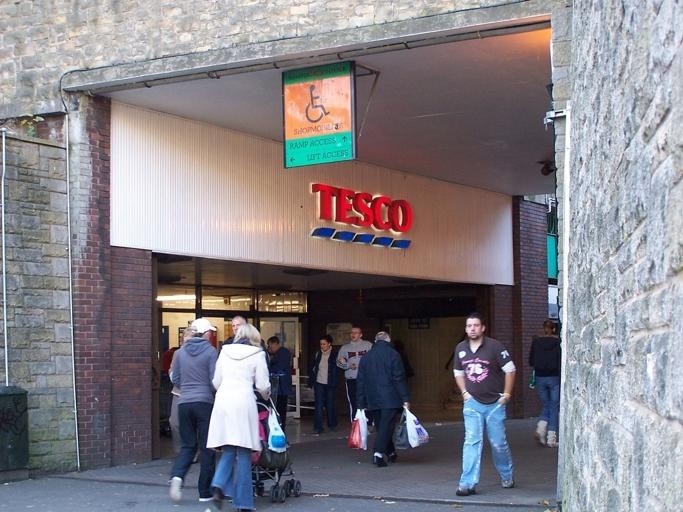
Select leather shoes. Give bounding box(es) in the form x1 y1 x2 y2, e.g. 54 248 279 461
388 452 397 463
373 456 387 466
213 487 231 502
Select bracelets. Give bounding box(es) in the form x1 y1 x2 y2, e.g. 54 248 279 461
462 389 468 398
501 392 513 403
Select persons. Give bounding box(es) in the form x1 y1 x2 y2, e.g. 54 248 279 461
336 324 375 427
168 328 201 464
355 331 412 467
205 323 272 512
528 320 561 448
169 318 219 504
222 315 246 344
267 336 294 433
452 312 517 496
307 334 346 436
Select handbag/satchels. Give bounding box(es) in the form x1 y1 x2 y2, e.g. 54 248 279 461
258 441 288 469
528 369 536 390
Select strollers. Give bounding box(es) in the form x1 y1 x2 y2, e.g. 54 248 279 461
247 390 301 502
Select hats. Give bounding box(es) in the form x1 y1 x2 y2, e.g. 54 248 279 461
191 319 216 334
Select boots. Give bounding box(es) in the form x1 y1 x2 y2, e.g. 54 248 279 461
533 420 548 446
547 431 556 448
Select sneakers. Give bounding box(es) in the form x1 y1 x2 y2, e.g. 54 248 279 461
502 474 514 488
170 476 182 501
456 488 475 496
199 494 213 502
